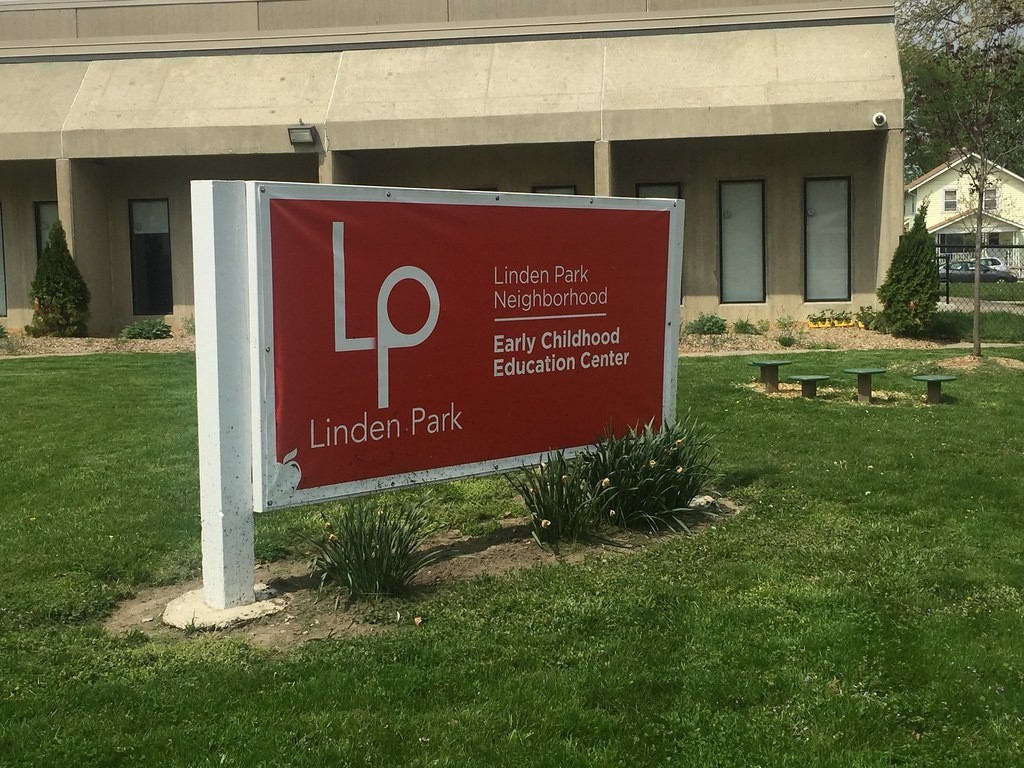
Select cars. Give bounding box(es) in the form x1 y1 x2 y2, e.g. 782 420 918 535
938 259 1018 284
958 256 1009 273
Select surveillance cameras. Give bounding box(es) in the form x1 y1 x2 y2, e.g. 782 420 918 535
872 112 886 126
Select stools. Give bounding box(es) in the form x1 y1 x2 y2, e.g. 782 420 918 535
747 359 792 393
843 368 886 403
912 375 957 405
788 375 830 398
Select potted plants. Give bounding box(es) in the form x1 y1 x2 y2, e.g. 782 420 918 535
806 305 874 327
